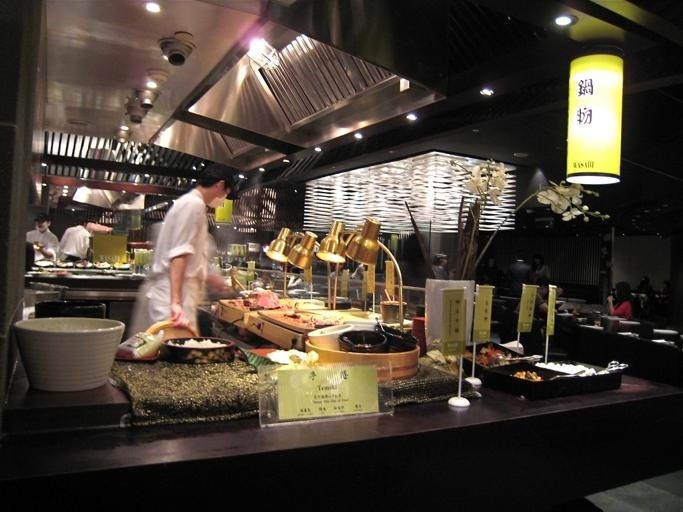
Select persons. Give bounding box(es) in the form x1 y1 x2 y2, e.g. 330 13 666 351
432 254 450 280
534 254 551 280
330 260 345 280
59 217 90 262
656 280 674 308
26 213 59 264
607 282 633 319
635 276 655 297
129 163 237 342
513 281 563 353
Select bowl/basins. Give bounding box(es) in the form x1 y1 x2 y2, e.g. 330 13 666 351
307 320 419 353
161 336 235 364
12 316 125 394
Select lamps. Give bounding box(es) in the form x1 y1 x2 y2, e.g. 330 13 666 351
266 219 405 330
567 30 624 187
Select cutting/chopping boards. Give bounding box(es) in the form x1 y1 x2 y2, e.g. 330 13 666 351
259 310 333 333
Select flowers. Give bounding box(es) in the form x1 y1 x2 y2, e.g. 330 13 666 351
449 156 610 275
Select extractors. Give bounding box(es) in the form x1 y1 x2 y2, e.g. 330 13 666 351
249 32 412 137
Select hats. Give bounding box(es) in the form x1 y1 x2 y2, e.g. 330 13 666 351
34 212 53 223
204 163 241 201
